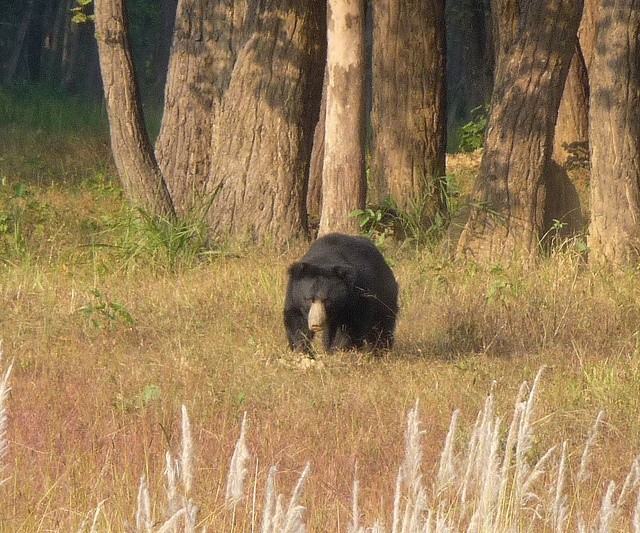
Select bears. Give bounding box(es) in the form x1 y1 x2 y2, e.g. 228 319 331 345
282 232 399 362
